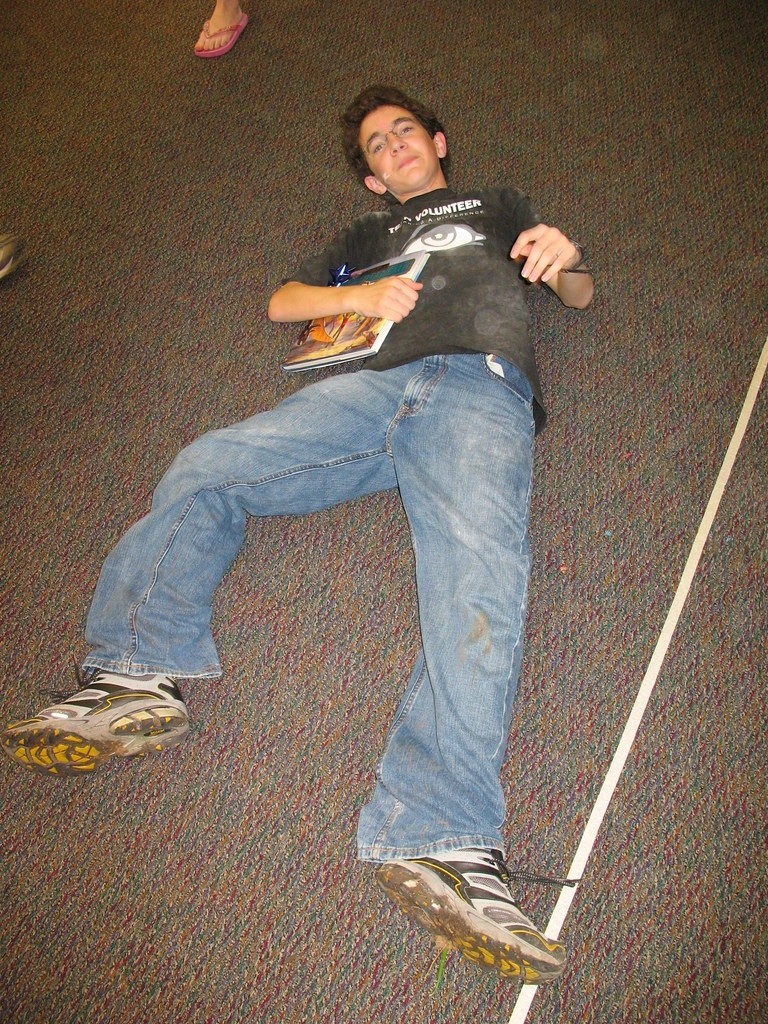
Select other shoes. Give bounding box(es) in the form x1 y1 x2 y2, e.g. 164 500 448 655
0 233 23 279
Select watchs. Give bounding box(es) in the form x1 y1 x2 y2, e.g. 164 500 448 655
560 237 593 273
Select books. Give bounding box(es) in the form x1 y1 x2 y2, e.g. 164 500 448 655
281 250 431 374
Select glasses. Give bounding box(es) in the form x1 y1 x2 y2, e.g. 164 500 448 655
360 116 420 157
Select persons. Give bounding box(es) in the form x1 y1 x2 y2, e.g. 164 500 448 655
2 86 595 982
194 0 249 57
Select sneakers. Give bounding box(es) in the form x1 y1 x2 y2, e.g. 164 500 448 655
376 847 583 984
1 651 190 776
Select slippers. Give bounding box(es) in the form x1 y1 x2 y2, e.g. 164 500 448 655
194 11 249 57
